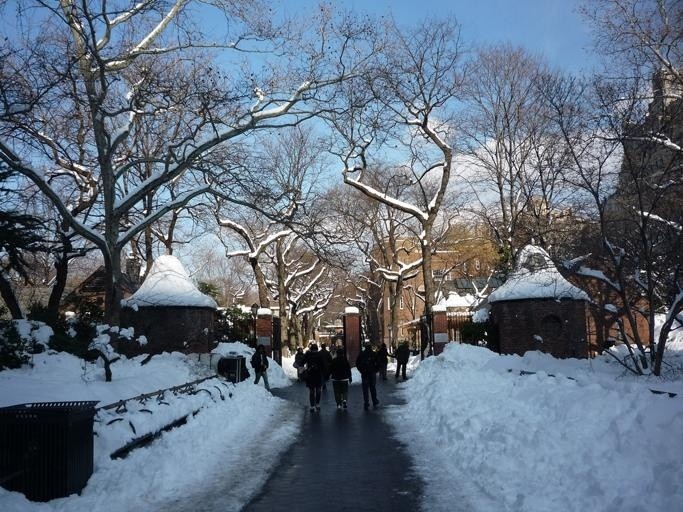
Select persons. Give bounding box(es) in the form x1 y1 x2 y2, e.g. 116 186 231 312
296 343 329 413
327 348 352 410
354 342 378 411
394 341 409 380
295 347 304 381
378 342 392 381
251 344 270 393
318 344 331 391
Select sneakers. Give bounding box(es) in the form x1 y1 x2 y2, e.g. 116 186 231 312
308 405 320 413
336 399 347 409
363 399 379 406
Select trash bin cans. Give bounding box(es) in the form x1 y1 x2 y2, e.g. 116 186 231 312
0 402 100 503
218 356 246 383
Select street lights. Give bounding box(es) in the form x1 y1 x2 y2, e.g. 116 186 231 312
251 303 259 345
426 310 433 355
386 323 393 353
